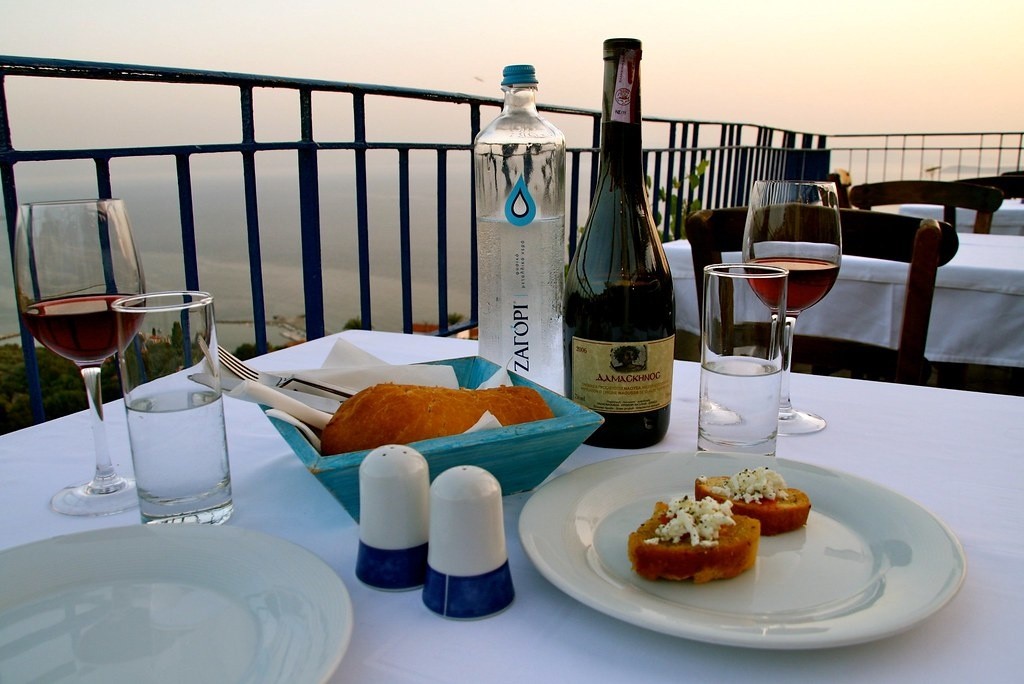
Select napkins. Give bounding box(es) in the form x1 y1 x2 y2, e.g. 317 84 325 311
201 335 516 452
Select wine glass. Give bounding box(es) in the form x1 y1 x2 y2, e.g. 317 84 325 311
14 198 146 517
742 181 842 437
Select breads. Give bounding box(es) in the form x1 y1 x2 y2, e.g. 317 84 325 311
628 501 761 583
321 382 554 456
694 476 812 535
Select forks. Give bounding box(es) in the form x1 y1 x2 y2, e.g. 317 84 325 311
218 346 358 399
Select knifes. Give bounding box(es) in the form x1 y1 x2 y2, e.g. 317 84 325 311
188 374 341 414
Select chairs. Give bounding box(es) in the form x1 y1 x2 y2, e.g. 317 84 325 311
684 162 1024 382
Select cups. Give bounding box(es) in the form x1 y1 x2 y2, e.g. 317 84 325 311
112 291 234 524
698 263 789 457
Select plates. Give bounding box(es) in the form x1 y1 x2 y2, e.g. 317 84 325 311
0 524 353 684
519 451 966 649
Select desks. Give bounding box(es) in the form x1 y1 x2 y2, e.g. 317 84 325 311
1 330 1023 681
663 226 1022 368
898 193 1023 236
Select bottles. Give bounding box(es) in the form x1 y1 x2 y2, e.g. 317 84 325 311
562 39 675 450
474 65 566 395
